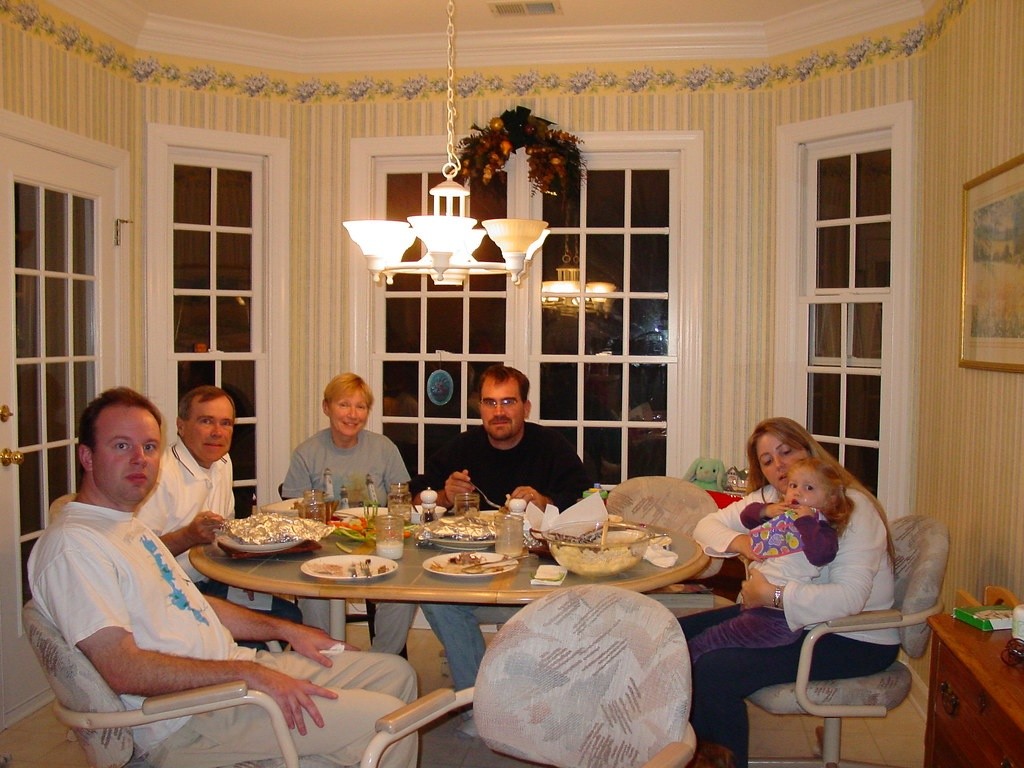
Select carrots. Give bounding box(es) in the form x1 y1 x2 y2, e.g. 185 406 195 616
328 517 411 538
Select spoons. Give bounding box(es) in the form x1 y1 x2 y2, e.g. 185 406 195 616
469 480 501 508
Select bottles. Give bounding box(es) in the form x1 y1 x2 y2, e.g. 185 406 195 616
387 482 411 531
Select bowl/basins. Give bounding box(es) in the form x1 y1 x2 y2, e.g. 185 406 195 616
411 505 448 525
542 521 656 577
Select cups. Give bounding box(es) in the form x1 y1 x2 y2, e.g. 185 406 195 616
376 514 404 558
455 493 480 520
494 514 524 557
302 488 326 524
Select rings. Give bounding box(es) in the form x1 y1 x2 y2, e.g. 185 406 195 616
529 493 535 498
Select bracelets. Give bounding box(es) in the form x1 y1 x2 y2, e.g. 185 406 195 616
773 586 781 608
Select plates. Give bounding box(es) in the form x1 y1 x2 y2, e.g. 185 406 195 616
431 539 494 550
301 555 399 581
216 535 321 561
334 507 388 519
422 552 519 577
649 532 672 547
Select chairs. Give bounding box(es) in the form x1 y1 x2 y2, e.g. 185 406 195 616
19 597 300 768
609 476 953 768
357 582 698 768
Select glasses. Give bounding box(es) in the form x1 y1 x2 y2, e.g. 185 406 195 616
477 398 518 409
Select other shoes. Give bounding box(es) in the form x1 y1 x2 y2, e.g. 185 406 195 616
456 706 481 739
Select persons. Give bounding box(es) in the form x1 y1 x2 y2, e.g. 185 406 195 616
280 373 411 659
686 457 849 662
27 387 419 768
133 383 304 651
411 364 588 692
678 417 901 768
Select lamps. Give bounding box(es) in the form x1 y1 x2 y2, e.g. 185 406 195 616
337 0 548 290
539 183 620 318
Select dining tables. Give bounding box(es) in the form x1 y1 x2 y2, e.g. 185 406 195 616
187 512 709 654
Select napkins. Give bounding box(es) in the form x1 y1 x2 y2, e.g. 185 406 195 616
643 544 678 570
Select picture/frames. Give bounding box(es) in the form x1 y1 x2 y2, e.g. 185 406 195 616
956 153 1024 373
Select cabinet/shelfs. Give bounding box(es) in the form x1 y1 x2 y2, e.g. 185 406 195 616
924 614 1024 768
